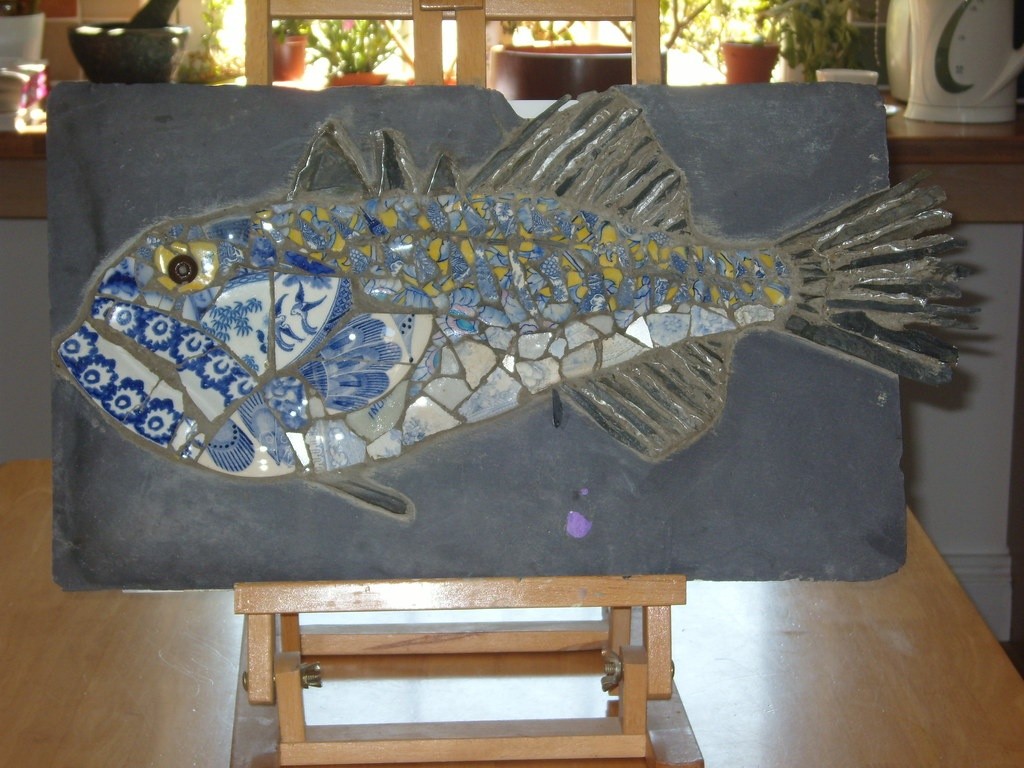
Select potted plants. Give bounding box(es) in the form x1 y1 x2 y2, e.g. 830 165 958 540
271 17 312 83
309 16 396 89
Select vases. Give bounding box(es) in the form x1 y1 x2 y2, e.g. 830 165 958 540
722 40 783 86
490 44 667 100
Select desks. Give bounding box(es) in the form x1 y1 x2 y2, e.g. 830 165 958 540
1 457 1024 767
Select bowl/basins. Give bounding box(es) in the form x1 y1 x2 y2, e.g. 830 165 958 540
68 23 191 83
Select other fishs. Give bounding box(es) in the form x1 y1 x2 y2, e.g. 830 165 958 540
51 84 970 524
